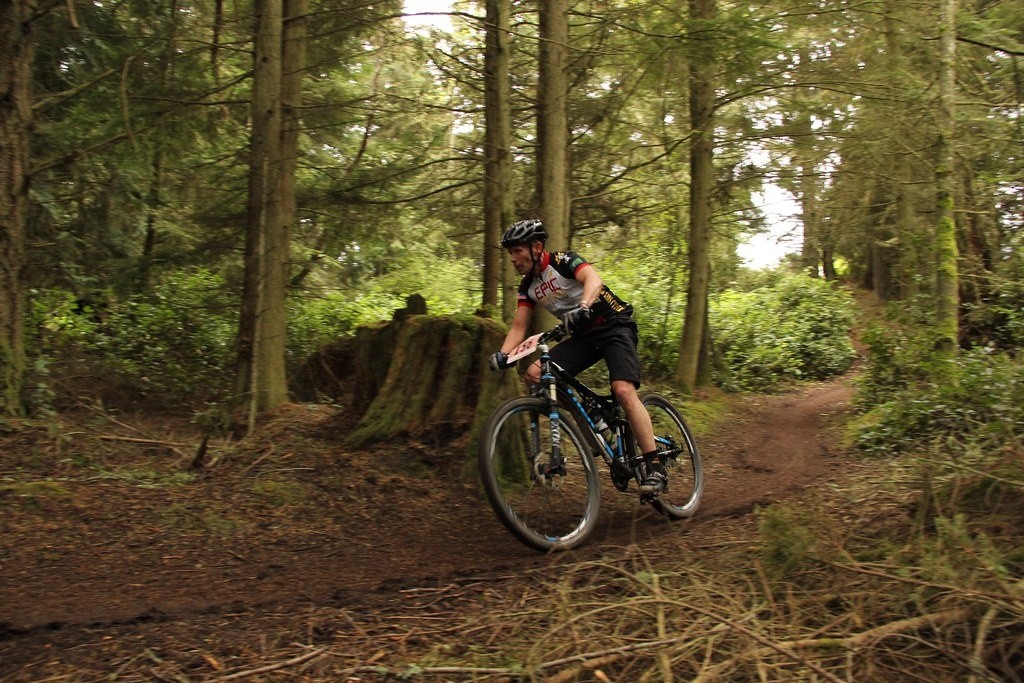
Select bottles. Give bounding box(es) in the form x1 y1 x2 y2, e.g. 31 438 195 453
590 413 617 446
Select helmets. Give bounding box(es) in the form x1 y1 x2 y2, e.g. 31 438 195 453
501 219 549 247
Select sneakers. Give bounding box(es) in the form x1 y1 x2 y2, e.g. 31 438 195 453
639 468 671 491
592 402 618 457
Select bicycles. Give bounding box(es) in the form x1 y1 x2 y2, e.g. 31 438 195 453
477 345 703 553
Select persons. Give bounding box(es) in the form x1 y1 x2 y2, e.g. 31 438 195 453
488 221 668 496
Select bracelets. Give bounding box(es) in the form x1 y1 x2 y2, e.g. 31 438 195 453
577 300 590 307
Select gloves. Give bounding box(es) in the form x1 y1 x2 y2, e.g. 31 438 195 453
490 352 510 373
564 308 590 335
